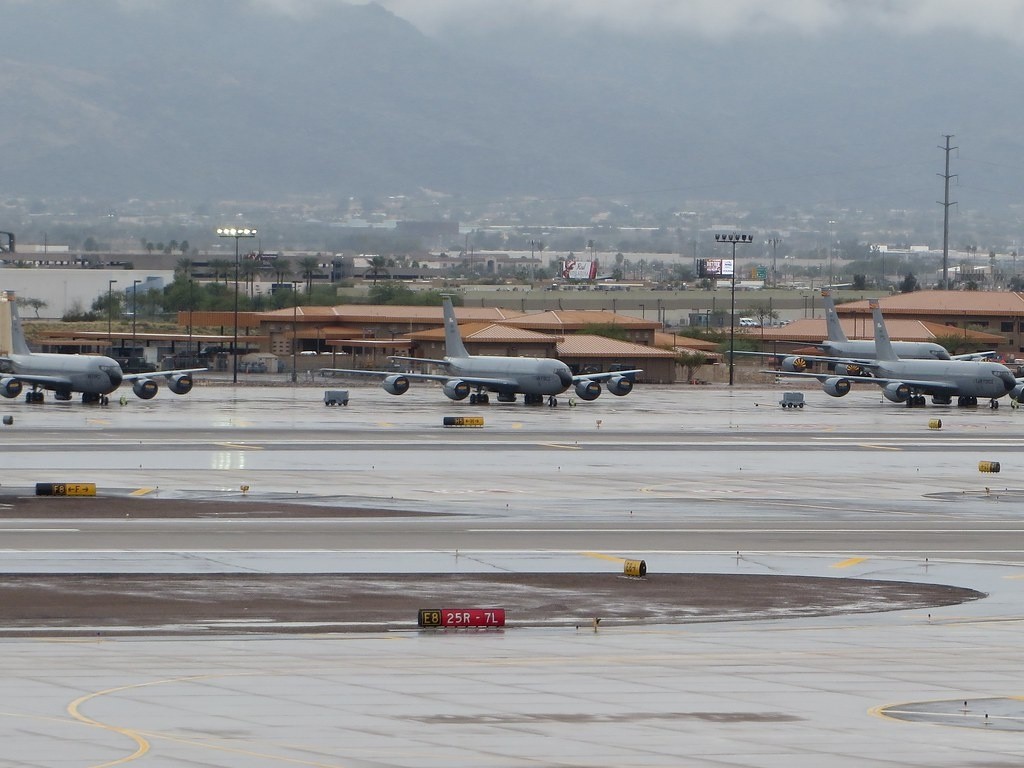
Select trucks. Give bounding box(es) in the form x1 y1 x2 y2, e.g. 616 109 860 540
115 356 157 374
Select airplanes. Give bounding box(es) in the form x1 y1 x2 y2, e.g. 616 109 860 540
319 294 644 408
759 297 1024 409
727 286 996 383
0 302 207 406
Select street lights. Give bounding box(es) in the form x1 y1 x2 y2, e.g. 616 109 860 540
109 279 117 341
292 280 303 374
715 233 754 385
218 227 258 381
134 281 142 351
526 239 541 290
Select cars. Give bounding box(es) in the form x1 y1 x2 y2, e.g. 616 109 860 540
200 345 220 356
238 357 268 373
160 351 208 370
740 318 758 327
